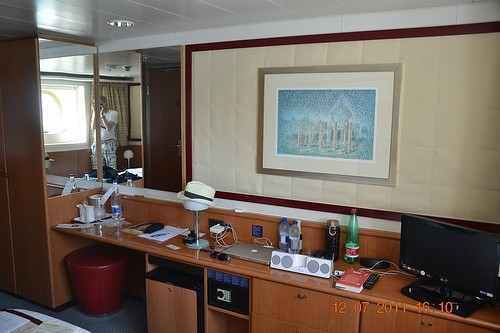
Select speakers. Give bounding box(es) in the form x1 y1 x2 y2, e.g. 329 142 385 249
270 251 333 278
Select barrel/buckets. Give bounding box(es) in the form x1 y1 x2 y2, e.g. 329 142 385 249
63 245 128 315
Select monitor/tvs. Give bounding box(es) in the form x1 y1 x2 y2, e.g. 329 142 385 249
398 214 500 318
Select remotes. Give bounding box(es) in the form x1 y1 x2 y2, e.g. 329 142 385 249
363 274 380 289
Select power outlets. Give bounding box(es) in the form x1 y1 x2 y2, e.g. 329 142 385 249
208 219 223 232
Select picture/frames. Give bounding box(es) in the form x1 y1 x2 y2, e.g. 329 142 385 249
256 62 403 187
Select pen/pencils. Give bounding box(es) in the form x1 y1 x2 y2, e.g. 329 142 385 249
201 249 217 252
149 232 171 239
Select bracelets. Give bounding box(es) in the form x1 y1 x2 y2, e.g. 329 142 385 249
101 115 105 118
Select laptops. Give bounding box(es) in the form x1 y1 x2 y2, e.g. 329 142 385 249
223 243 287 265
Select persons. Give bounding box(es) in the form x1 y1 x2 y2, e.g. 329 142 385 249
91 96 118 171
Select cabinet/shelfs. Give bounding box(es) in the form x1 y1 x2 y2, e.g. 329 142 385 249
144 253 251 333
360 302 500 333
0 38 97 310
251 277 361 333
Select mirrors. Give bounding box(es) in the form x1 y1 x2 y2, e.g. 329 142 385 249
38 35 99 199
99 45 183 193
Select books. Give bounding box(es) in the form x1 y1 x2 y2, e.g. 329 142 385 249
336 270 371 294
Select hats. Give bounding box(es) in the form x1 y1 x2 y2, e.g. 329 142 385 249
176 181 216 207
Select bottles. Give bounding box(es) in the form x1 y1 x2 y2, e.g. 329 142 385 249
89 194 106 217
278 218 291 252
343 209 359 264
324 219 341 261
126 177 136 187
110 180 123 219
287 221 300 254
69 173 78 192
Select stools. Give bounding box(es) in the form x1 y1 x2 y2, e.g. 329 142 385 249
65 247 125 316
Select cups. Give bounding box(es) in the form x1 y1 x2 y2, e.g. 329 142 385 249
79 205 95 223
